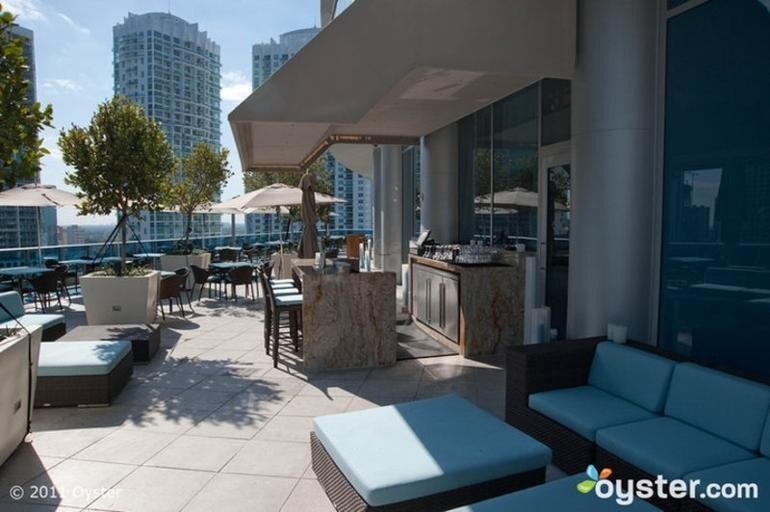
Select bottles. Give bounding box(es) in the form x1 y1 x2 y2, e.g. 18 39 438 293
359 241 365 269
457 239 493 264
365 246 371 272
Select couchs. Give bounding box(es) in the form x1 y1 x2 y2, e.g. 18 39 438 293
442 469 666 512
507 335 770 511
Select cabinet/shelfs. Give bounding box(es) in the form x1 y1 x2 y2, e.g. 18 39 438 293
407 251 526 356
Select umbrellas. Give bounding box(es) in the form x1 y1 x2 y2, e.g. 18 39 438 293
160 199 243 250
226 206 340 217
297 172 319 259
208 183 349 256
0 183 90 266
73 188 165 256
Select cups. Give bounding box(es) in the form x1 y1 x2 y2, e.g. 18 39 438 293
315 252 320 264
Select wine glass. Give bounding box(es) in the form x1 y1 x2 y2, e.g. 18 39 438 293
421 244 460 261
